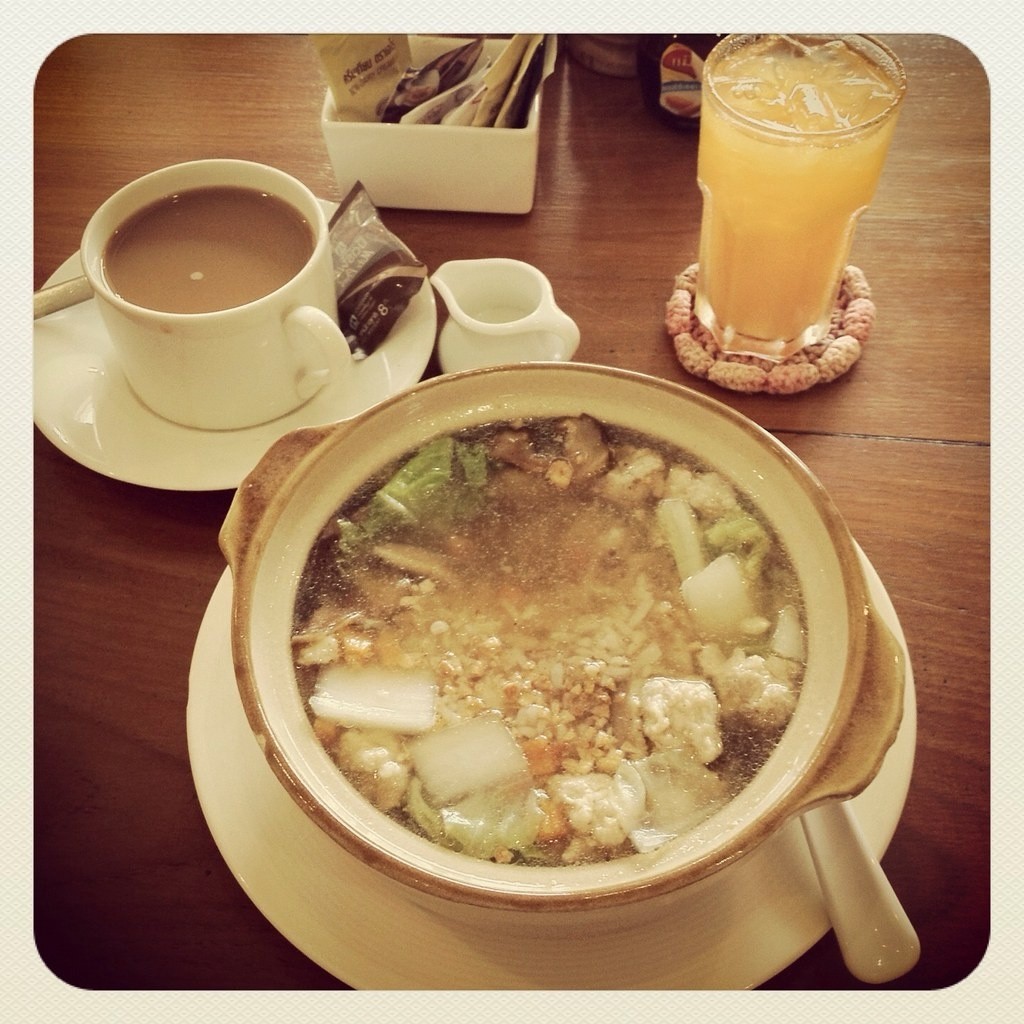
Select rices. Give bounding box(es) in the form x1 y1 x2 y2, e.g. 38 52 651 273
368 523 688 777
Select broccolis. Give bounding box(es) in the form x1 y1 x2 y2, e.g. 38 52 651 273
341 444 804 858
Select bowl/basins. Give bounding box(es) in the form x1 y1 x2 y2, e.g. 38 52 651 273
215 363 911 934
319 34 541 216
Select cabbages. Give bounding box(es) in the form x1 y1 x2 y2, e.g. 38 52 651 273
294 434 771 853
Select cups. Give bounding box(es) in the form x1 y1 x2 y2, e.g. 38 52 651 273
430 258 580 379
696 32 908 363
79 159 356 433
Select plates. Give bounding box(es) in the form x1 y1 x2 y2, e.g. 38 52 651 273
35 196 438 491
183 537 917 991
